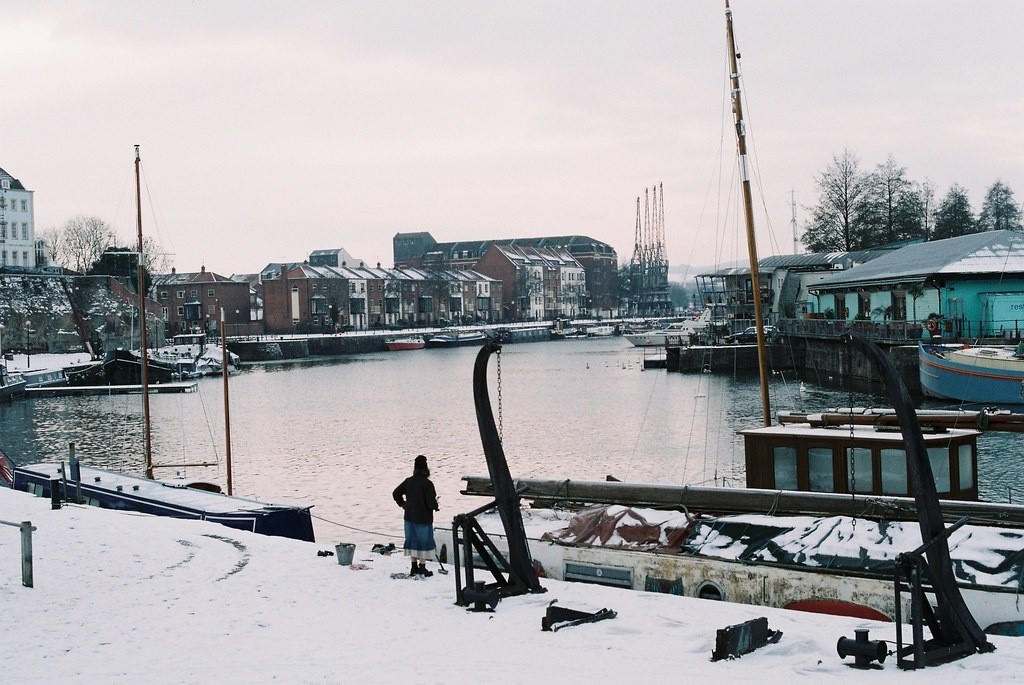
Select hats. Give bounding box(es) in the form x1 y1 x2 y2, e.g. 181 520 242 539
414 455 430 477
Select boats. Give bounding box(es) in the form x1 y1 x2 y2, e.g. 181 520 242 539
503 328 552 343
429 332 487 347
62 349 175 386
623 320 727 347
133 327 240 372
13 459 316 542
385 335 426 351
552 318 663 341
918 340 1024 403
432 476 1023 638
21 367 69 388
0 364 27 401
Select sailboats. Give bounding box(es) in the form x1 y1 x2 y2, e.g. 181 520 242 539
132 144 226 495
608 0 1024 502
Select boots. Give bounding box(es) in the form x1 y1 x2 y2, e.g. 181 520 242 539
411 562 420 574
419 563 433 577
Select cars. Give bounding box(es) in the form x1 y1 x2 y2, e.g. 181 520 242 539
730 325 781 343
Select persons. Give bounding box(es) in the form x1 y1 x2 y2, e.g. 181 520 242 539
90 333 105 359
393 455 439 577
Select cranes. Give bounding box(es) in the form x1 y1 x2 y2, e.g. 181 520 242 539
630 181 672 309
